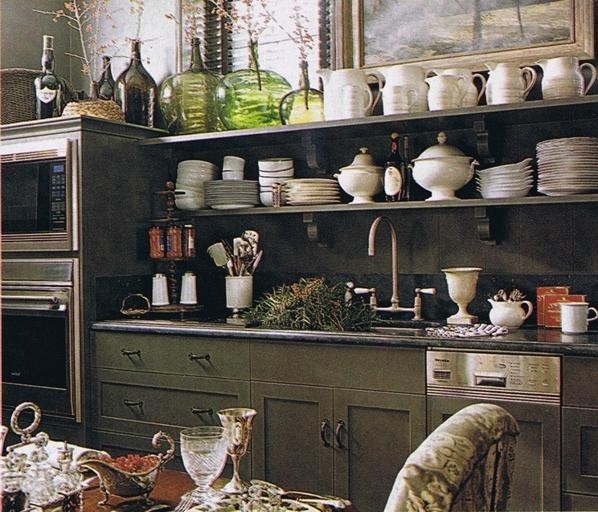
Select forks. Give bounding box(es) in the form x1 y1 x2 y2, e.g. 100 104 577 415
166 494 196 512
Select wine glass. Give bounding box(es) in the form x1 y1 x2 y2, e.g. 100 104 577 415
441 268 484 324
179 426 234 503
217 407 257 493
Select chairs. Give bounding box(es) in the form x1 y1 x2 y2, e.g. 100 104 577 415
383 403 521 512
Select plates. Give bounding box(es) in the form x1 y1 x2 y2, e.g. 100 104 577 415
474 158 534 199
284 178 342 206
203 179 259 210
535 137 598 196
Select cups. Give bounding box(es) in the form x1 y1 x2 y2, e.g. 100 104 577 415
559 302 598 335
224 276 253 308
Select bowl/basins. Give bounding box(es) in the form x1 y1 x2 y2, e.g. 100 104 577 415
257 157 295 207
173 159 220 210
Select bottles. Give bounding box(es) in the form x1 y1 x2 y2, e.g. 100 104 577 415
34 34 62 120
280 61 324 125
383 132 411 202
98 39 159 129
157 37 223 136
2 438 80 511
151 274 170 306
179 273 198 304
213 41 294 131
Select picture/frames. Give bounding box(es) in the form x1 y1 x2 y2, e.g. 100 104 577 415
317 0 595 91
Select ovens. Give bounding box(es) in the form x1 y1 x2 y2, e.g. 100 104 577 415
0 261 76 421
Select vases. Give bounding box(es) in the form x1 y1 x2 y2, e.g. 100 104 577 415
90 38 324 136
487 287 534 329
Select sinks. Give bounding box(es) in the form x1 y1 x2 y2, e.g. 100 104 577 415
354 319 443 334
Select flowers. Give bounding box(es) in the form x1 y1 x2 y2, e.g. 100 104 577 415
32 0 315 110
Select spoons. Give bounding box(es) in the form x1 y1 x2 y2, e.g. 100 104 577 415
247 479 353 509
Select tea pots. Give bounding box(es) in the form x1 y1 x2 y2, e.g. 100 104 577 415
316 56 596 122
487 299 533 330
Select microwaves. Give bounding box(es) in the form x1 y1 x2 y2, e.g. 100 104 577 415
0 138 79 254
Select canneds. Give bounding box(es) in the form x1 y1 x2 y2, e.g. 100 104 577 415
272 183 281 208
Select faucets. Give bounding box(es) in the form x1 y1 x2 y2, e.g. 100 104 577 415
368 214 400 310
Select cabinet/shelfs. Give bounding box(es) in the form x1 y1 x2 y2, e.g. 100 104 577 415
88 328 598 512
